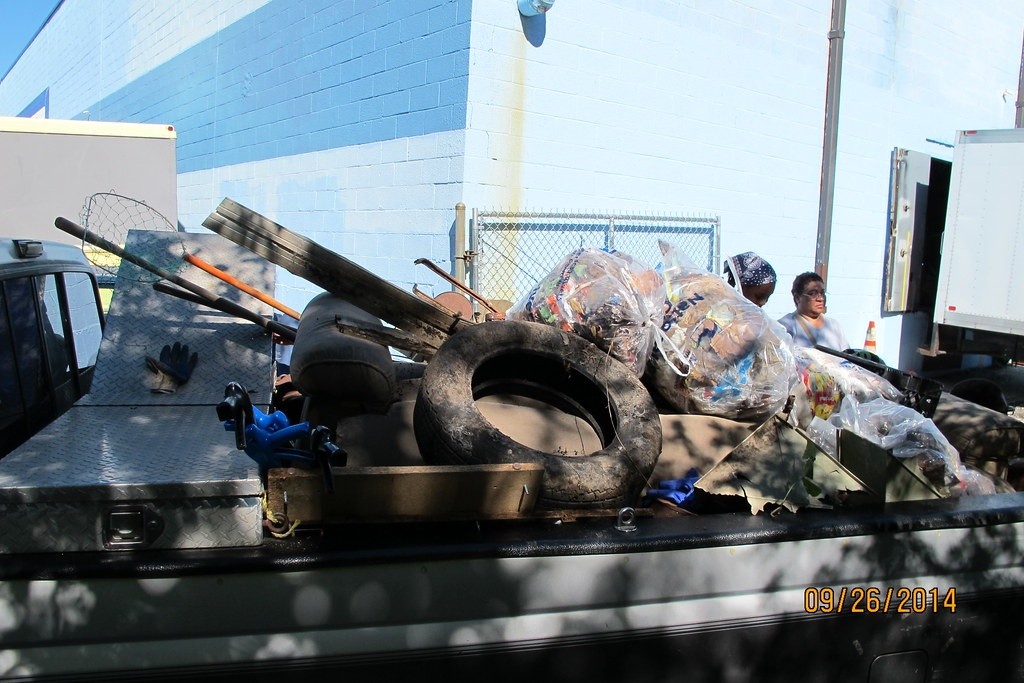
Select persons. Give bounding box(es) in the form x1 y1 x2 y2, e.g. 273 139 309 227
724 252 776 308
776 271 850 352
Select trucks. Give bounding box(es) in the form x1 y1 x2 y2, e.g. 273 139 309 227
1 128 1024 683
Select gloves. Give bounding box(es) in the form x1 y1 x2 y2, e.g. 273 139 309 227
146 342 198 394
